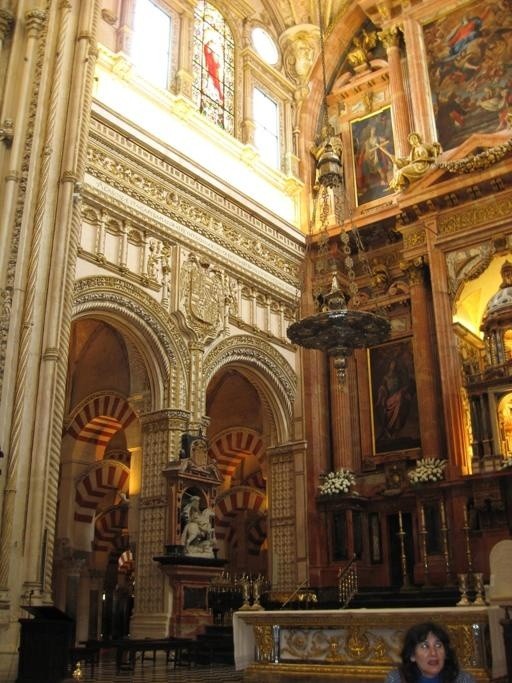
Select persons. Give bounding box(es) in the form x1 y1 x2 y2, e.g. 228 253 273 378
181 492 218 549
352 123 398 189
375 351 415 438
427 4 511 141
378 131 444 192
387 623 473 683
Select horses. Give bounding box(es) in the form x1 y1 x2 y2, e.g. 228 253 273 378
181 506 218 555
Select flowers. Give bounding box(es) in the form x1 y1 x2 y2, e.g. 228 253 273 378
407 455 448 484
319 464 362 495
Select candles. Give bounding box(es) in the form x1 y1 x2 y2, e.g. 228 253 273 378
394 498 469 533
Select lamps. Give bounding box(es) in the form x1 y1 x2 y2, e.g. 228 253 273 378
280 0 394 393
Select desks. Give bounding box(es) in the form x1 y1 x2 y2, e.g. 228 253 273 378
232 604 508 682
207 589 270 625
77 636 221 673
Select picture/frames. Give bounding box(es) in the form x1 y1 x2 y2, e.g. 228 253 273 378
416 0 512 152
348 103 399 207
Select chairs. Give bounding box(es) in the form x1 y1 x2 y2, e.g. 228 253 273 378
482 538 512 623
